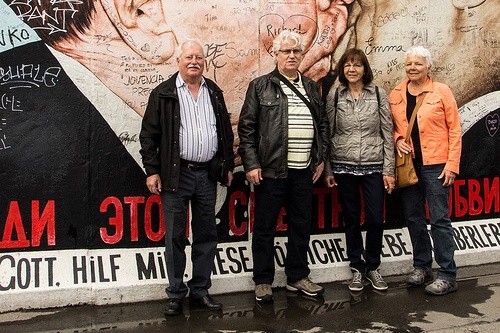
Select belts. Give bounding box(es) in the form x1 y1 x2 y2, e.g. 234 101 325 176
179 160 213 171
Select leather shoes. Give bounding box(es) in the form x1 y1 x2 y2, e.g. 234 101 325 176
164 298 183 314
189 295 223 309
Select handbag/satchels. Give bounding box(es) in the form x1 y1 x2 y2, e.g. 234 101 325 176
394 147 419 187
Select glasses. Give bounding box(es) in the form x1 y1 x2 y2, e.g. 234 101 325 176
279 48 303 56
343 63 364 69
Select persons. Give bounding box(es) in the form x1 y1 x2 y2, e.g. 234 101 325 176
139 41 236 317
387 47 461 295
325 48 395 291
237 30 329 300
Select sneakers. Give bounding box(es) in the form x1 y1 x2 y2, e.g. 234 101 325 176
425 278 459 295
349 267 364 291
364 268 389 290
254 284 274 300
285 275 324 296
406 267 433 284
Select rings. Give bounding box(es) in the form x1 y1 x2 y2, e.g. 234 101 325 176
394 185 395 186
452 180 454 181
